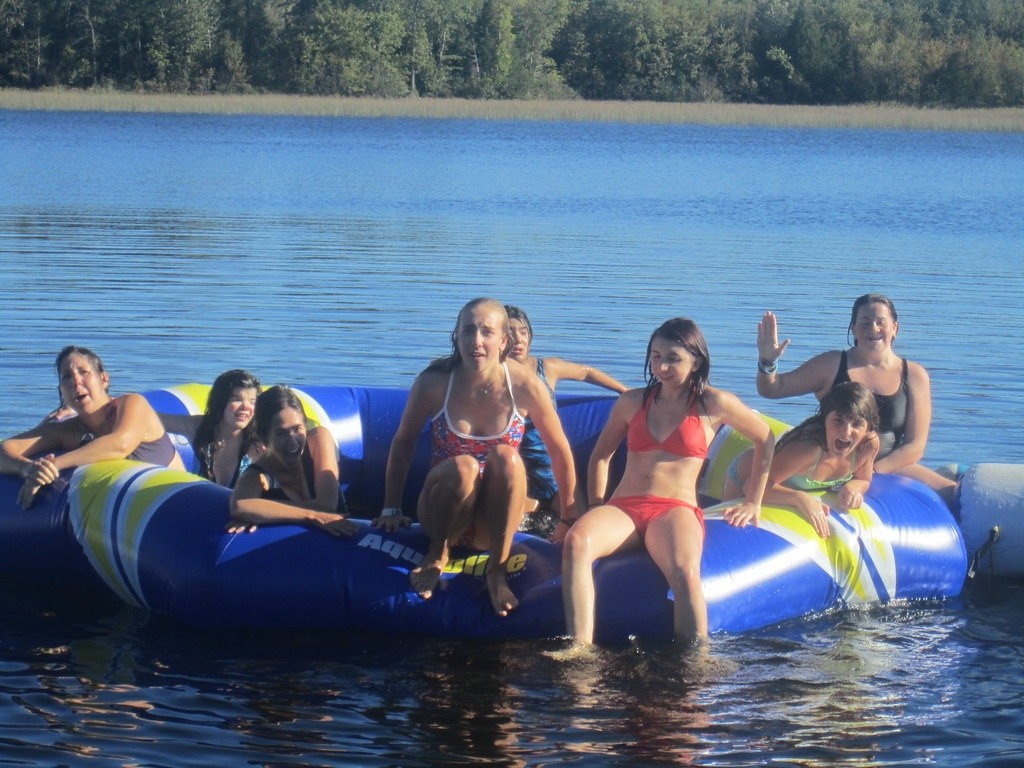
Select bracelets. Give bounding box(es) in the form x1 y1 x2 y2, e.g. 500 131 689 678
758 360 777 374
588 503 605 507
379 507 403 519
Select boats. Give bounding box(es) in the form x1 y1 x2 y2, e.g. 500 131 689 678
1 383 967 643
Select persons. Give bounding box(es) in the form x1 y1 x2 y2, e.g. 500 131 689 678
755 293 961 508
222 382 360 539
0 344 187 512
541 314 777 683
502 304 637 539
369 297 584 619
721 379 881 540
46 369 270 491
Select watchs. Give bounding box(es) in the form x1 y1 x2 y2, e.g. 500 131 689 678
560 517 577 527
